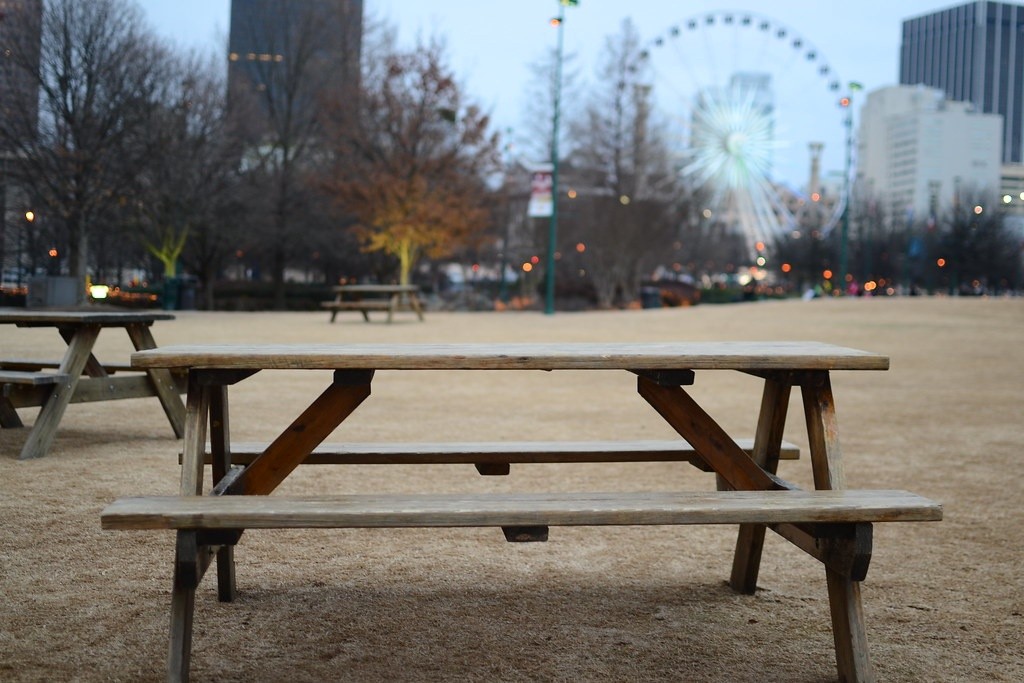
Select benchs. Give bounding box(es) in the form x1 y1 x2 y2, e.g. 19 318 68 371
0 356 189 395
398 299 430 311
99 489 944 586
0 371 71 410
322 300 392 312
174 436 802 476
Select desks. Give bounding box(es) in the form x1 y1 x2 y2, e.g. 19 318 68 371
330 284 426 326
0 309 185 461
129 340 890 683
